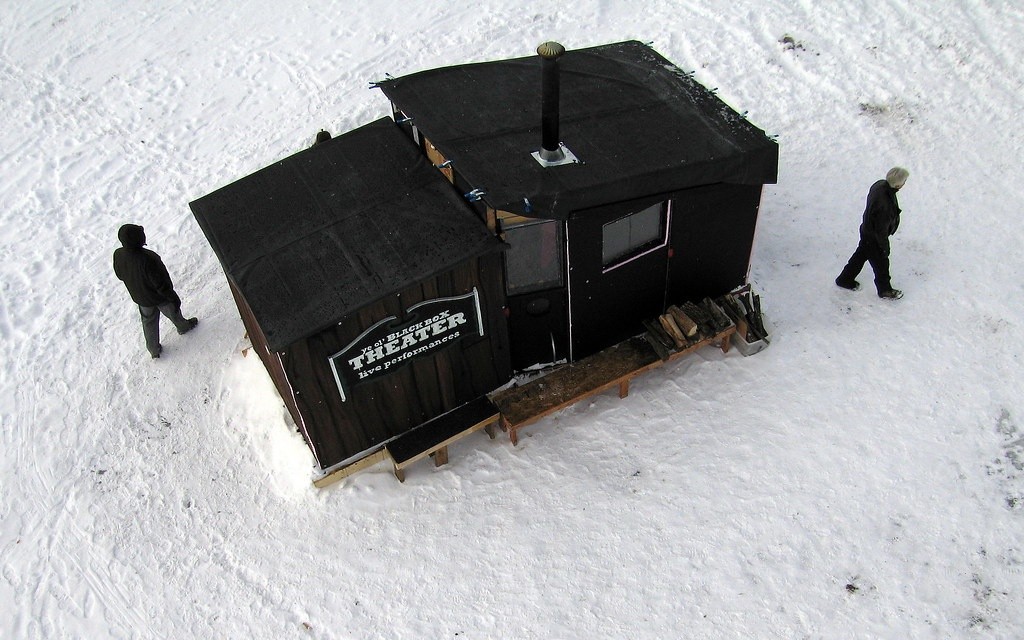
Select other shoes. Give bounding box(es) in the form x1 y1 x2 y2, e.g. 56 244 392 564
881 289 904 300
849 282 861 291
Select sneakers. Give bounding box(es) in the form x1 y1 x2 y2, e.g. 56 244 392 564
178 317 198 335
152 344 162 359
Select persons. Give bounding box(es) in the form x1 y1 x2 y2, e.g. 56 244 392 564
113 224 198 358
835 166 909 300
